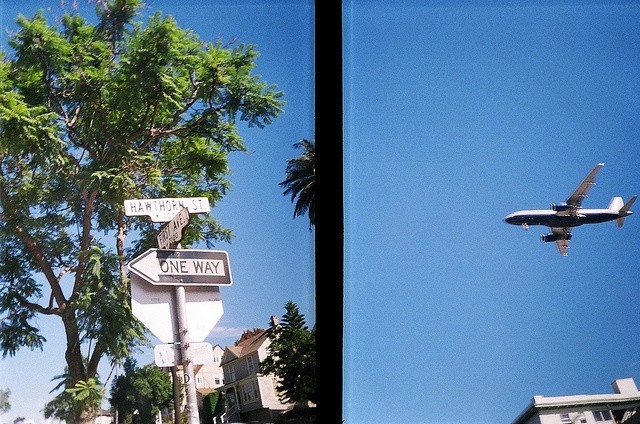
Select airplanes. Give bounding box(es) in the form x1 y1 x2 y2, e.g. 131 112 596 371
503 164 634 257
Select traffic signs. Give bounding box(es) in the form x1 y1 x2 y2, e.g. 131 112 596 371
124 196 210 222
128 249 233 287
156 208 191 251
